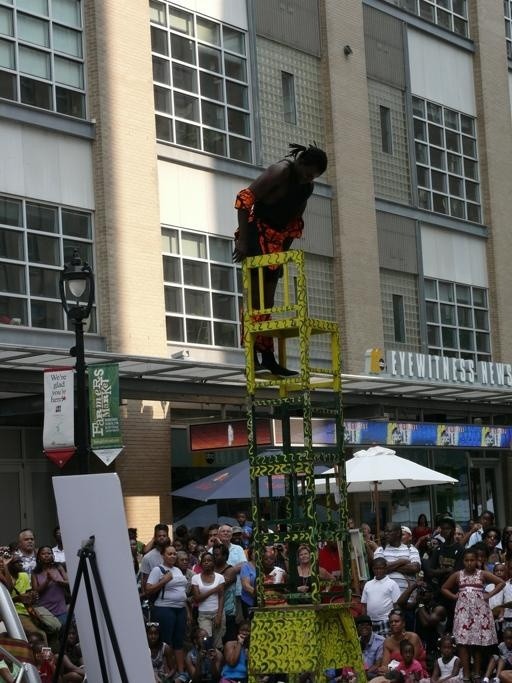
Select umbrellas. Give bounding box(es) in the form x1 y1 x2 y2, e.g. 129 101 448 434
168 448 332 504
296 443 460 547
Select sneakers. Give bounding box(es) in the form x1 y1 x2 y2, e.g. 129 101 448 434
262 362 299 377
244 364 272 376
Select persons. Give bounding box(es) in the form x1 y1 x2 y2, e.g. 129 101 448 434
231 141 328 376
1 512 86 683
125 512 511 682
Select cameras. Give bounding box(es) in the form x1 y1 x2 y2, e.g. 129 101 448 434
3 551 11 558
477 528 484 535
417 585 431 602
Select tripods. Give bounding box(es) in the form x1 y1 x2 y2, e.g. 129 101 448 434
54 535 129 683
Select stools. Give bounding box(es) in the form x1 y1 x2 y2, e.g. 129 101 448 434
248 608 368 683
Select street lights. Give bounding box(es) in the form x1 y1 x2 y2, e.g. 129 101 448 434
55 254 112 472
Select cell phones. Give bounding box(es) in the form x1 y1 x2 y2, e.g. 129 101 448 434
202 636 214 655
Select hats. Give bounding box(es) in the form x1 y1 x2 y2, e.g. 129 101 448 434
232 526 243 534
401 526 412 536
